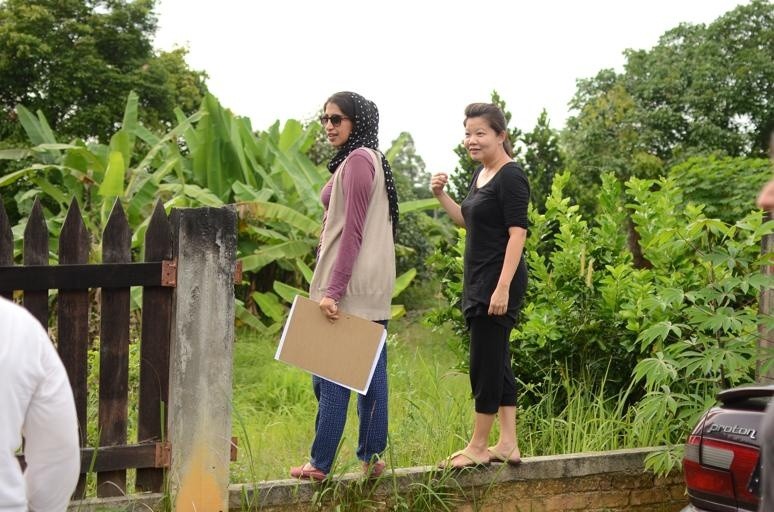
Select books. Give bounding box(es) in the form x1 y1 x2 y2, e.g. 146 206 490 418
274 294 388 396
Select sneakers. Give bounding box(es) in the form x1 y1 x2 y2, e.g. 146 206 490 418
289 463 329 484
362 458 387 481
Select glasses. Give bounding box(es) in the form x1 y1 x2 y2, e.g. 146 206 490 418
319 111 355 128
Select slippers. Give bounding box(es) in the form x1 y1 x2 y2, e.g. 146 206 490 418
435 448 493 470
487 444 523 468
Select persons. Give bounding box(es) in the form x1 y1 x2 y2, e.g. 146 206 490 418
431 103 531 471
0 296 82 512
289 91 399 482
